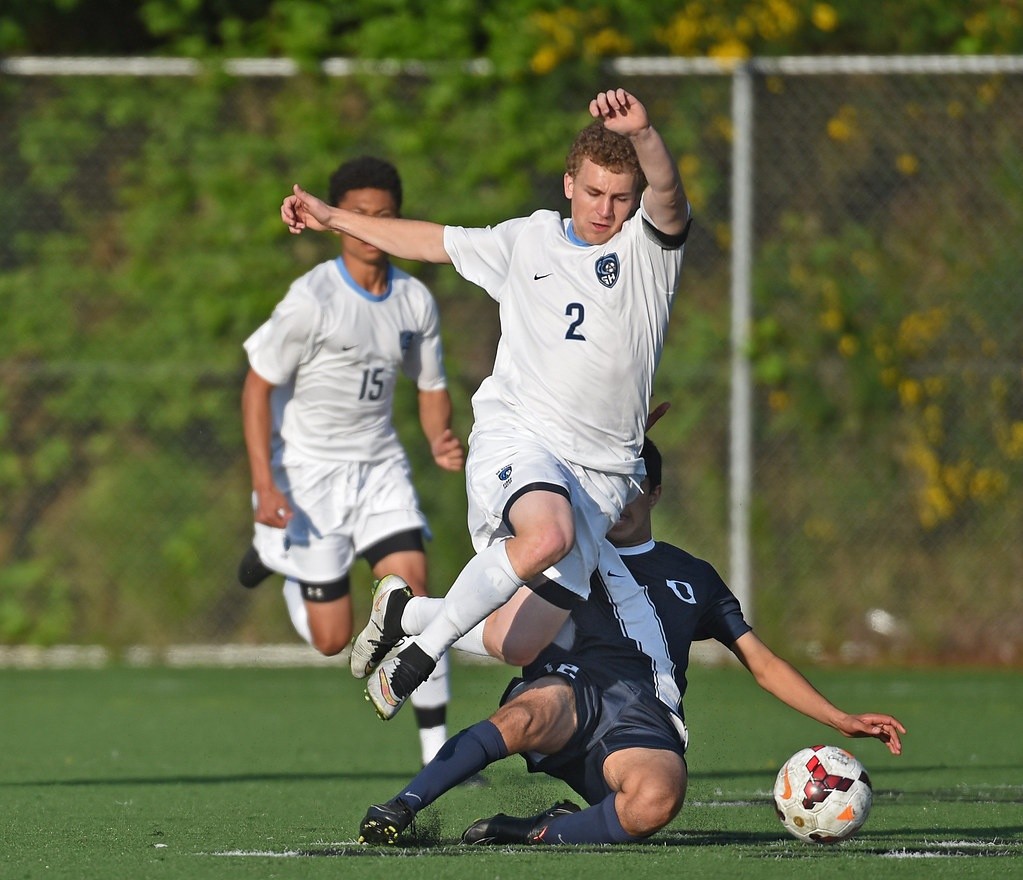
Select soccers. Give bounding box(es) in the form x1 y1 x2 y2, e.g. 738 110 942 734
772 745 873 842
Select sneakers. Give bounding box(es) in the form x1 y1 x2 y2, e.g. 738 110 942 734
238 536 290 589
365 657 408 721
360 799 417 846
461 799 582 846
350 574 415 680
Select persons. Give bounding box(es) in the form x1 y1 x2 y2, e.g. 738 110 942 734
358 393 909 846
236 154 494 792
280 88 694 720
